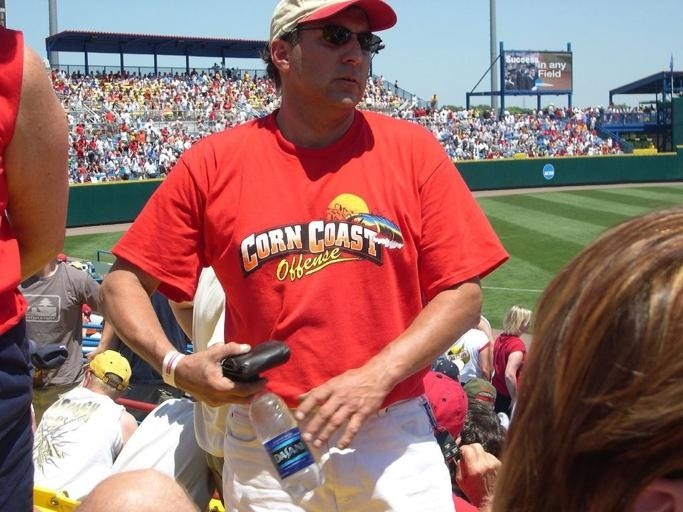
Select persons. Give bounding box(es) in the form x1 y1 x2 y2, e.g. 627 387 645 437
0 24 71 512
18 253 534 512
488 201 683 510
97 0 512 511
44 61 682 187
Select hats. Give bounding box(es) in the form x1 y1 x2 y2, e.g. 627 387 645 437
424 359 497 435
90 351 131 392
269 0 397 41
57 254 85 269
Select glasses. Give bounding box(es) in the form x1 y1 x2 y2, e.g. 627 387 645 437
289 24 385 54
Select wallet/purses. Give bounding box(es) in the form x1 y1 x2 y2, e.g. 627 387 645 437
225 342 289 376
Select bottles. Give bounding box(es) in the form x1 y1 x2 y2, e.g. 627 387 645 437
247 387 326 502
